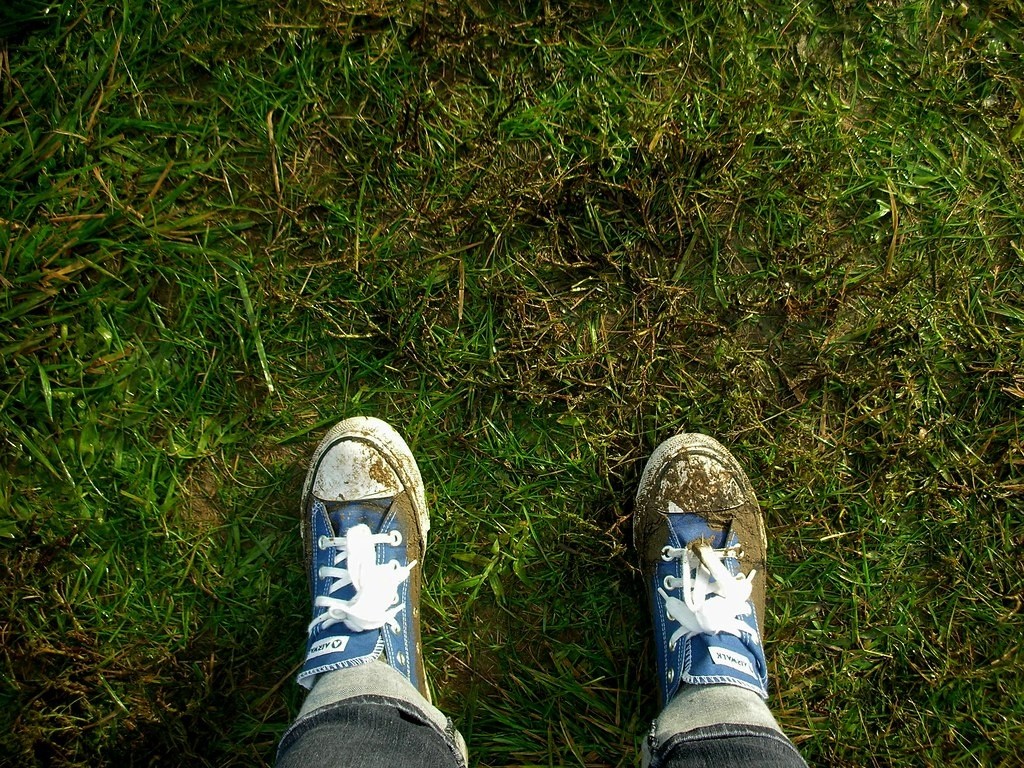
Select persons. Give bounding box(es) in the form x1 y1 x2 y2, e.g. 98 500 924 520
274 417 809 768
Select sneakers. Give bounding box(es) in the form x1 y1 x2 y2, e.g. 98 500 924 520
632 434 769 713
295 416 433 707
